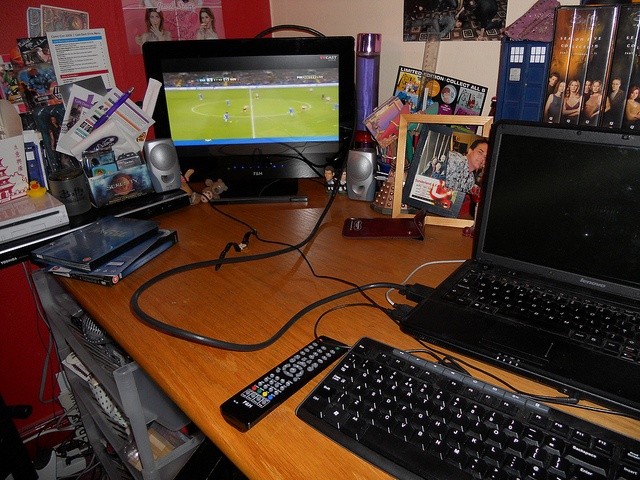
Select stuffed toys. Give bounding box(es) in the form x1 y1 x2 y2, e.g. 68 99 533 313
201 178 228 202
176 169 201 206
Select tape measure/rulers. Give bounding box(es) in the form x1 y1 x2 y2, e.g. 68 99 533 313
417 33 441 111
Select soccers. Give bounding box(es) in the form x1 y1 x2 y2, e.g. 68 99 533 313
230 120 233 124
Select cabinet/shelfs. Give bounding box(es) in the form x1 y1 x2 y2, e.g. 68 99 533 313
33 270 208 480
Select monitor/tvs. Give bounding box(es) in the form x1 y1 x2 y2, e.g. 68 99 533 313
142 35 355 204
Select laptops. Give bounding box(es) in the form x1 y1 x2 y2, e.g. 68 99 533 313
400 119 636 416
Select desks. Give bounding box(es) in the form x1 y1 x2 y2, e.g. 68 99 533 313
48 177 639 479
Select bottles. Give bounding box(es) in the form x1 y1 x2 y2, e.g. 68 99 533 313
355 33 381 146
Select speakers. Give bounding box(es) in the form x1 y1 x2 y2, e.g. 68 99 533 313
346 148 377 202
144 137 183 194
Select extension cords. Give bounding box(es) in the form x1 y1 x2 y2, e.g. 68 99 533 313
51 441 87 476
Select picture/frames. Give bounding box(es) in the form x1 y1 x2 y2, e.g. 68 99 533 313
390 113 494 228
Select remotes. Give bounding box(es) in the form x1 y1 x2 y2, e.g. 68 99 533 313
220 334 349 432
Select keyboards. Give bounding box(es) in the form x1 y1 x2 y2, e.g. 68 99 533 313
294 336 636 477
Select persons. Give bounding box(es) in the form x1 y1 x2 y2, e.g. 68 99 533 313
194 8 219 40
71 104 81 118
135 8 172 54
625 86 640 126
603 77 625 128
446 139 489 193
439 154 448 172
546 73 559 99
324 165 338 193
420 156 437 176
584 80 611 127
50 116 68 151
562 78 585 125
430 162 446 181
543 80 567 123
405 14 465 38
337 166 347 191
581 79 592 124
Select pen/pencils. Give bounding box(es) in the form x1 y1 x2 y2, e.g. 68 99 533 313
407 130 413 162
210 195 309 205
91 86 135 134
414 130 418 148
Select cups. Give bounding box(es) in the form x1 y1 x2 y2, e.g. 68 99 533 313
47 168 92 217
